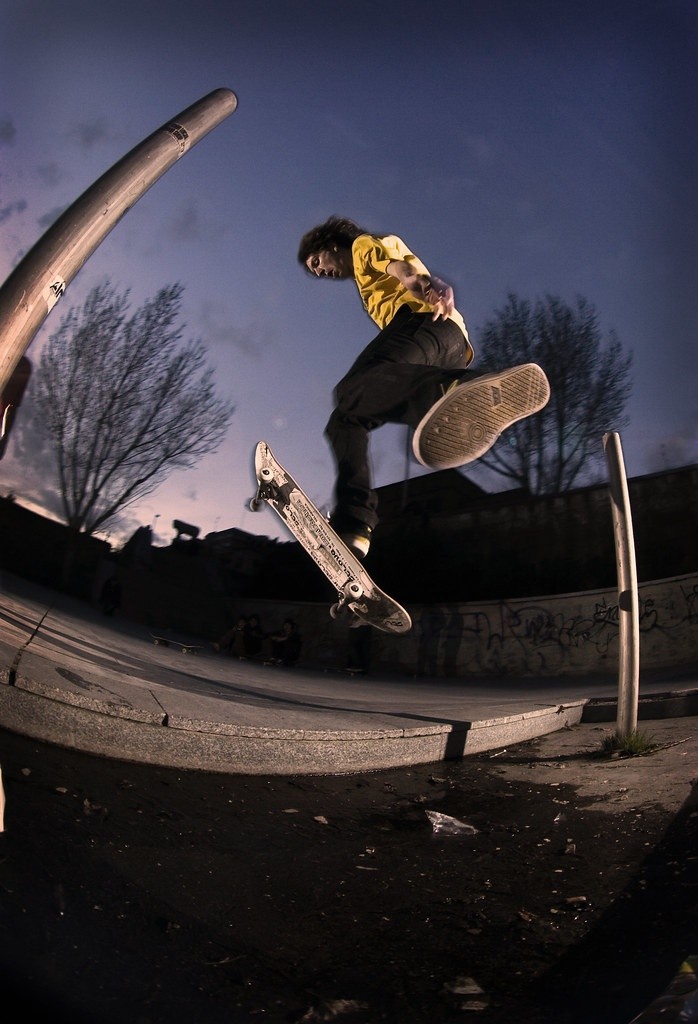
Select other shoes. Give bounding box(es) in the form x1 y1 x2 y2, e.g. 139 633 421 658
411 363 550 471
329 519 372 558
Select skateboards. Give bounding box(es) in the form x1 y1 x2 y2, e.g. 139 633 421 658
244 439 415 640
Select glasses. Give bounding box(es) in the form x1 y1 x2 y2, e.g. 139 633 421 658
311 246 326 275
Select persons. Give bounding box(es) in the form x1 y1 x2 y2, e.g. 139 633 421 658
297 213 550 557
